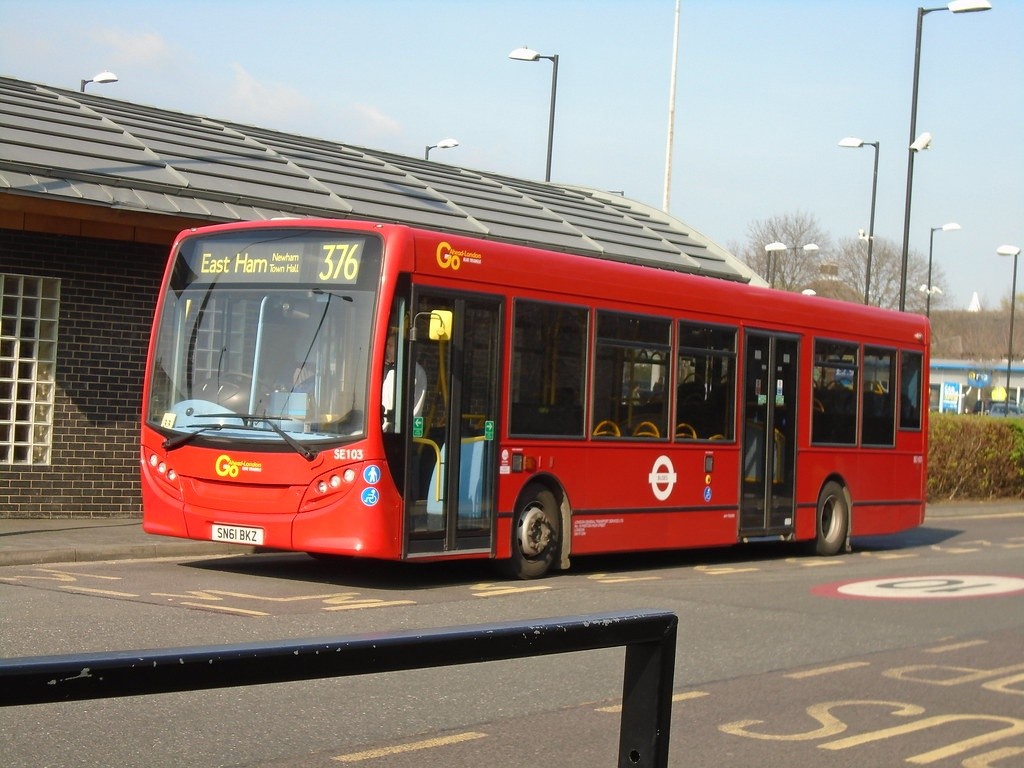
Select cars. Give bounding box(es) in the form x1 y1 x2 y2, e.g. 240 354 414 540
974 399 1022 418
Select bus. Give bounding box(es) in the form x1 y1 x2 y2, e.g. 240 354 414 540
138 216 931 581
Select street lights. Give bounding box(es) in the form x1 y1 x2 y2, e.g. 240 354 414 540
771 244 820 289
80 69 119 92
765 241 788 282
838 136 879 305
927 220 962 319
897 0 993 313
994 245 1022 418
424 137 460 161
507 46 560 183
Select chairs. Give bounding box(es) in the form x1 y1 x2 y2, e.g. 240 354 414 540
591 373 731 440
811 377 914 418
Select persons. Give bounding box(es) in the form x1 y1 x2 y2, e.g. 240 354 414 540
377 332 428 504
294 360 318 392
